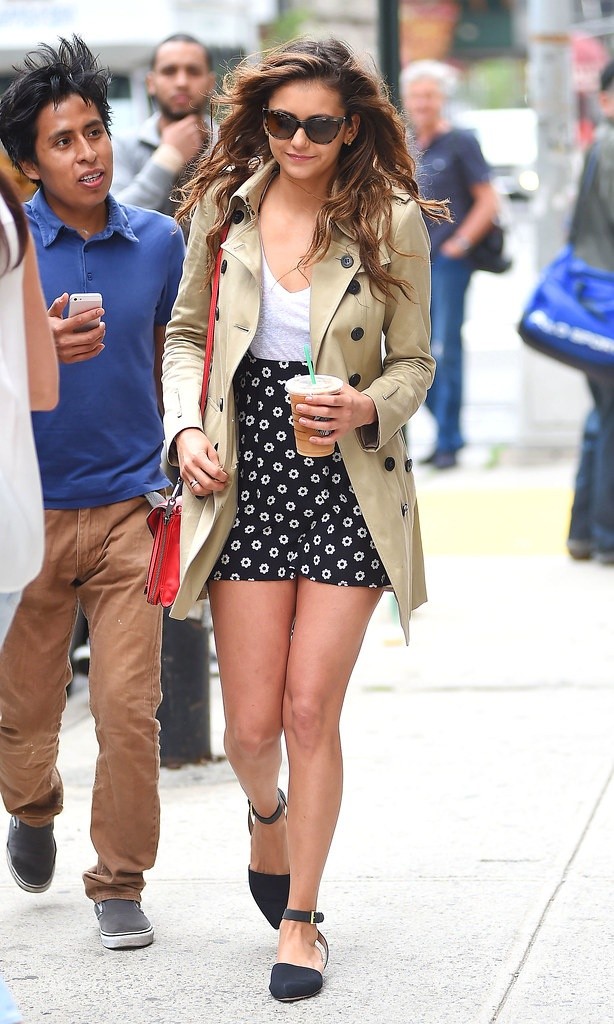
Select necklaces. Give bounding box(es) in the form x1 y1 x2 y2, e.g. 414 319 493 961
64 211 100 236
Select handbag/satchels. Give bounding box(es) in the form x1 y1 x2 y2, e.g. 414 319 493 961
518 247 614 368
474 220 513 275
144 494 187 607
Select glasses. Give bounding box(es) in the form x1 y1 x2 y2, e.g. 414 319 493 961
259 103 349 146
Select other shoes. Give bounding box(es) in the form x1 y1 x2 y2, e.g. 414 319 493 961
247 788 294 935
94 898 156 951
268 907 330 1004
3 810 59 895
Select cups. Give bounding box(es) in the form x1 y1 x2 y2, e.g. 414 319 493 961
284 374 344 458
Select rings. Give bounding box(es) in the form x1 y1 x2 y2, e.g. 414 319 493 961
189 480 198 488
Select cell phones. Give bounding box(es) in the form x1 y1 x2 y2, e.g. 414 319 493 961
68 292 103 334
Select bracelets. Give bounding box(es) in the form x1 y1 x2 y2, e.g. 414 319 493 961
452 238 475 253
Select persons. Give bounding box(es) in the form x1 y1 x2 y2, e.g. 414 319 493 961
566 62 614 569
391 59 502 463
0 31 187 950
103 33 221 237
156 36 436 1006
